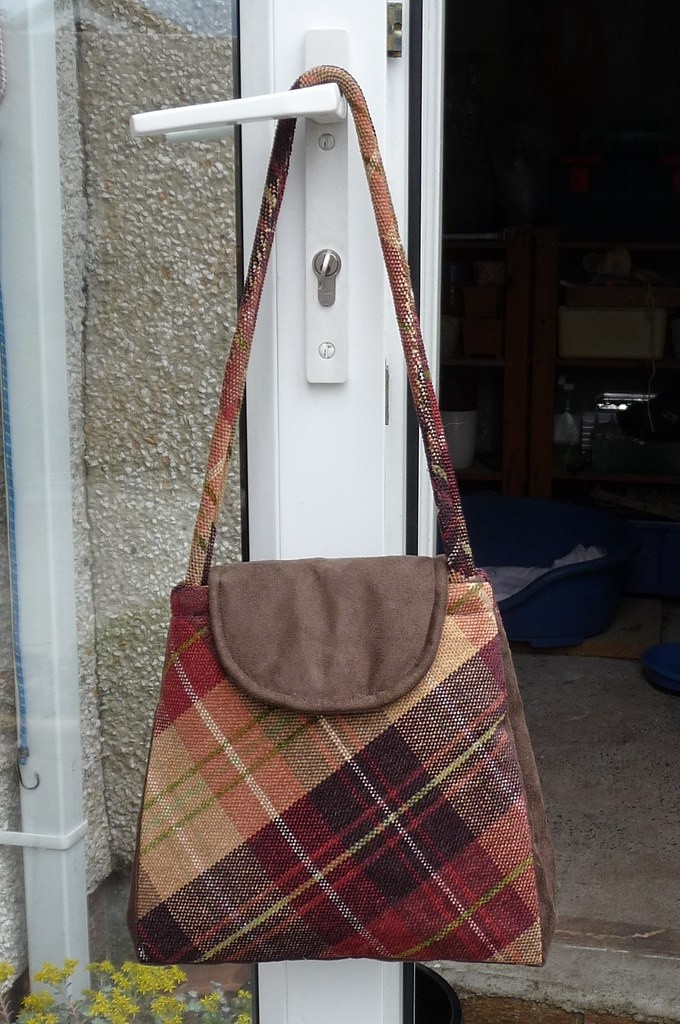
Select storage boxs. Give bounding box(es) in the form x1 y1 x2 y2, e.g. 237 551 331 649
560 307 667 363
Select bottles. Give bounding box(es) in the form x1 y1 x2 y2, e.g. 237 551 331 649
553 400 581 445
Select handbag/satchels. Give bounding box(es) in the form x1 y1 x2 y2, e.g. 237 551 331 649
127 66 556 966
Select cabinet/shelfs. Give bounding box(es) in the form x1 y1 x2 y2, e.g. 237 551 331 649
527 226 680 500
439 225 527 498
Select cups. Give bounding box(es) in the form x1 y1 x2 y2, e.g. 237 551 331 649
440 404 479 472
